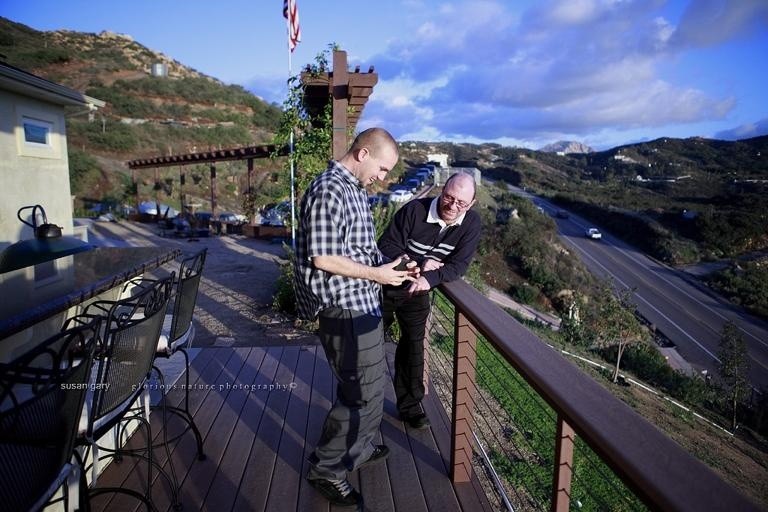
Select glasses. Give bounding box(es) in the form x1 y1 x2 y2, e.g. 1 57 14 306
442 195 468 209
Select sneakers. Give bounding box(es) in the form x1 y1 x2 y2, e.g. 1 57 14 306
307 465 363 509
398 403 431 429
361 444 391 464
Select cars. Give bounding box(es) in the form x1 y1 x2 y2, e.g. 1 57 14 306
555 209 570 219
585 227 602 240
366 162 435 211
219 213 243 223
254 201 290 227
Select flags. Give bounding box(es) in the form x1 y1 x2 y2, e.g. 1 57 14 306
278 0 302 53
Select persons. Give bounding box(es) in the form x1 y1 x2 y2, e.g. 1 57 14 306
288 125 422 512
372 171 483 433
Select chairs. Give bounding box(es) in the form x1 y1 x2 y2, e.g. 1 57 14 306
1 245 210 512
